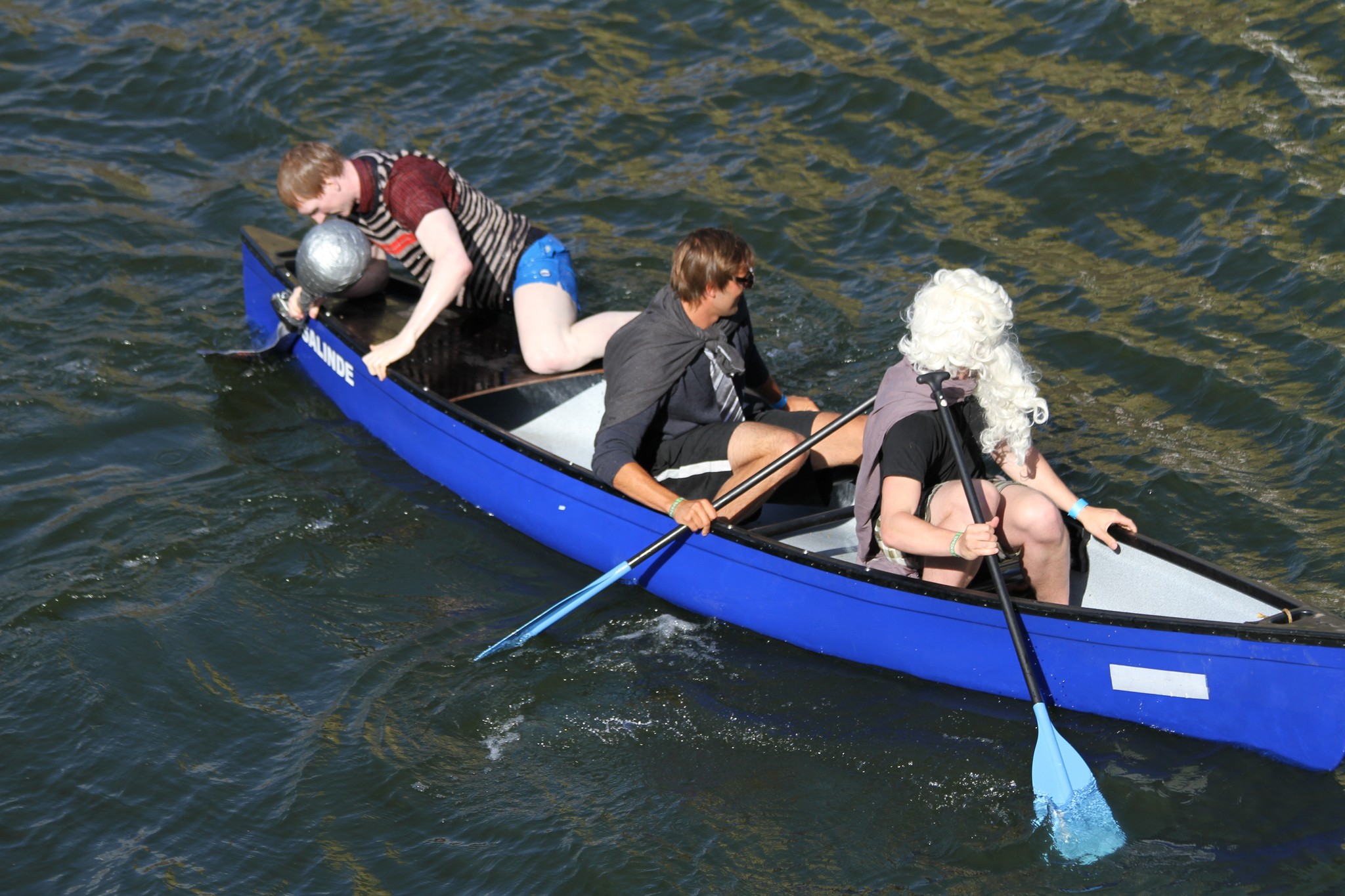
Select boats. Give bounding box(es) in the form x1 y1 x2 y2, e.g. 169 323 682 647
240 222 1345 774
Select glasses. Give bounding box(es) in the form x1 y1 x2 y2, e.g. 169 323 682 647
724 266 755 290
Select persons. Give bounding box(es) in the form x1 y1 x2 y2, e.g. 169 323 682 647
854 268 1137 605
276 141 641 381
772 393 787 410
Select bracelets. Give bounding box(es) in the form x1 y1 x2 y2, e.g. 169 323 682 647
949 532 963 558
669 497 686 517
1068 498 1089 519
590 228 869 538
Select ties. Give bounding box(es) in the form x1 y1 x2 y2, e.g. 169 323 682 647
704 345 746 424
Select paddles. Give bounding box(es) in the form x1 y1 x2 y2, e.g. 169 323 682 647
474 394 877 660
914 367 1128 869
